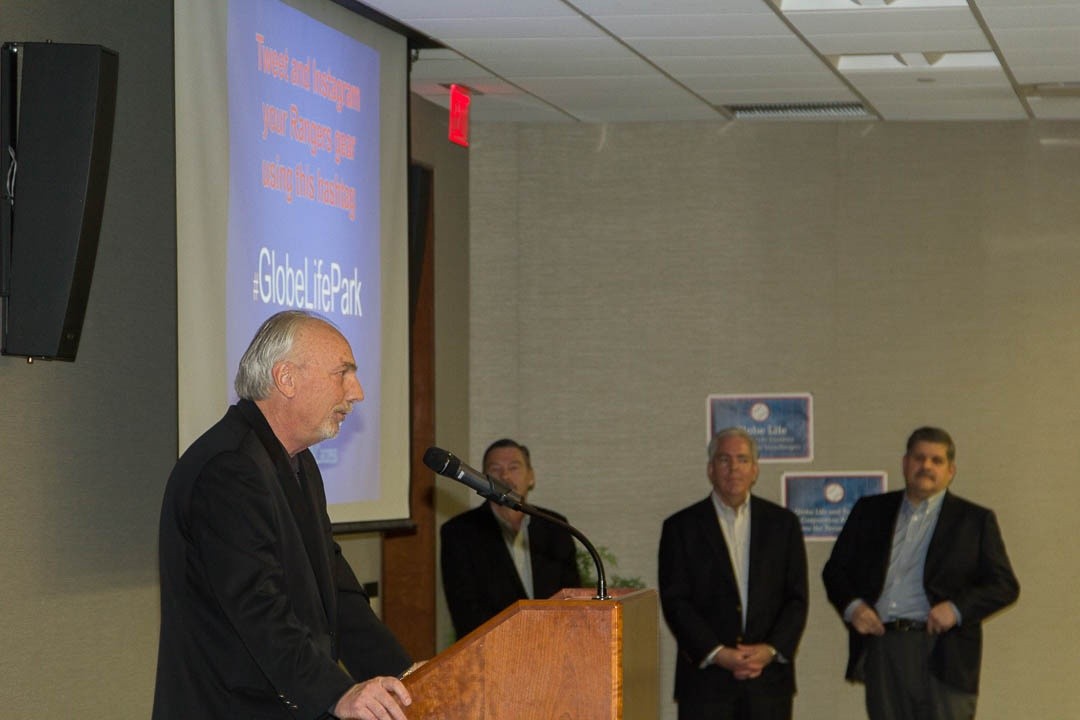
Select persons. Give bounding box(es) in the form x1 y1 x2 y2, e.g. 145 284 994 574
151 310 428 720
439 439 580 644
659 427 809 720
821 427 1020 720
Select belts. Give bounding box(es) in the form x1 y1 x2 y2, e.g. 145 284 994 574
883 618 926 632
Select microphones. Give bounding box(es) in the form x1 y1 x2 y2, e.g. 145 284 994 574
423 447 524 508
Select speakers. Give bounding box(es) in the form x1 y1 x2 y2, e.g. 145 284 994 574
0 38 119 362
